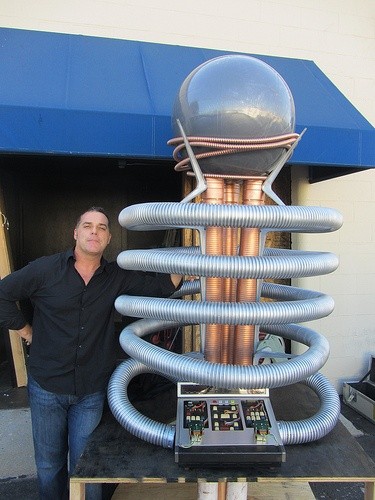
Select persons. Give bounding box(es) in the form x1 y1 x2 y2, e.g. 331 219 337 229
0 206 183 499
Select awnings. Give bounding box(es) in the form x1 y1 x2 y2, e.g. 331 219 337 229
0 26 375 184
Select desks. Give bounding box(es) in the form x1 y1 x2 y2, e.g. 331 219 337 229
64 381 375 499
